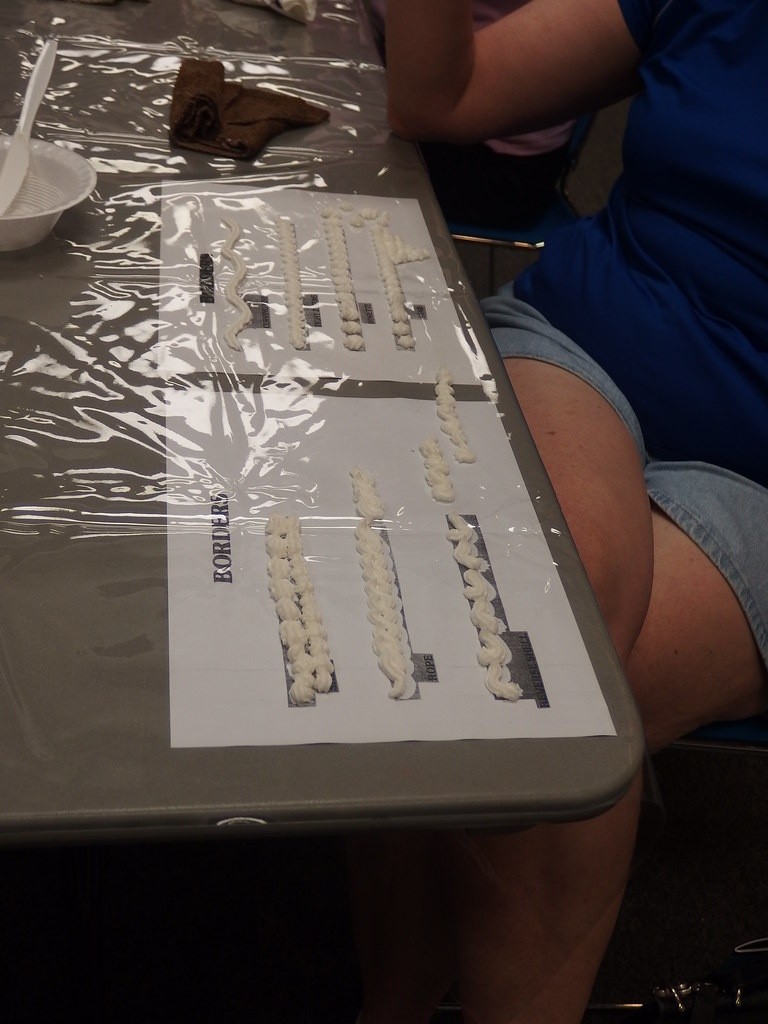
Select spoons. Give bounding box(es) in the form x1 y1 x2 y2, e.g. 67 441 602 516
0 40 58 217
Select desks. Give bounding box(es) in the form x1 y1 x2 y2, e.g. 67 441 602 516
0 0 646 1024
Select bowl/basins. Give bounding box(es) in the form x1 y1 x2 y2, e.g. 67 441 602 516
0 136 96 252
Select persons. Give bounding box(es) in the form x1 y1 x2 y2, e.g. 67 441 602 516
356 0 768 1024
370 0 572 228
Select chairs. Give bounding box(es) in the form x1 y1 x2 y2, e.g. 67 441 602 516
408 88 768 1024
